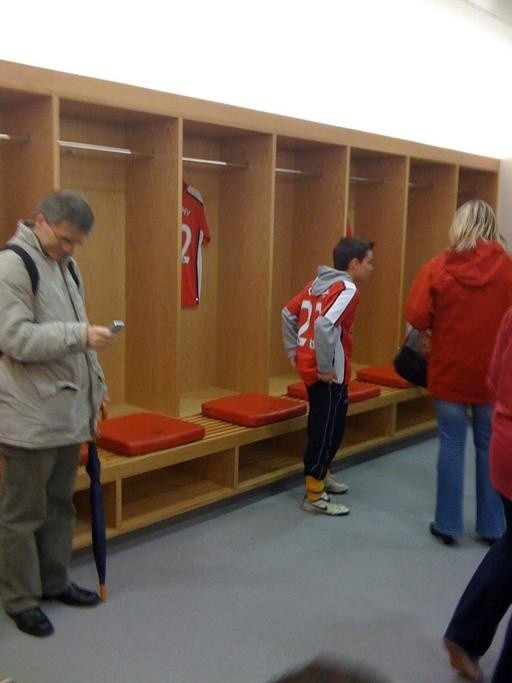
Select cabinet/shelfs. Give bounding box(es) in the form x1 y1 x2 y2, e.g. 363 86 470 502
0 59 501 552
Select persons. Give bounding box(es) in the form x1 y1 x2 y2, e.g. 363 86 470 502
405 198 511 547
0 188 117 637
441 309 512 683
281 236 375 517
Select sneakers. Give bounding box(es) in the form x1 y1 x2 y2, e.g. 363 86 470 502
443 637 484 682
301 491 350 516
323 477 349 495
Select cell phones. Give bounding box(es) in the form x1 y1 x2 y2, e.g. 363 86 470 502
112 320 125 333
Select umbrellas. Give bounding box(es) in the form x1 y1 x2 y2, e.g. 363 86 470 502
85 439 107 602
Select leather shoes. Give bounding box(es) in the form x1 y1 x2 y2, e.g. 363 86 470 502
38 582 100 607
5 606 54 636
430 521 454 544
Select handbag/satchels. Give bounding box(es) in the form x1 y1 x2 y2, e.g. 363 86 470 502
393 345 427 388
